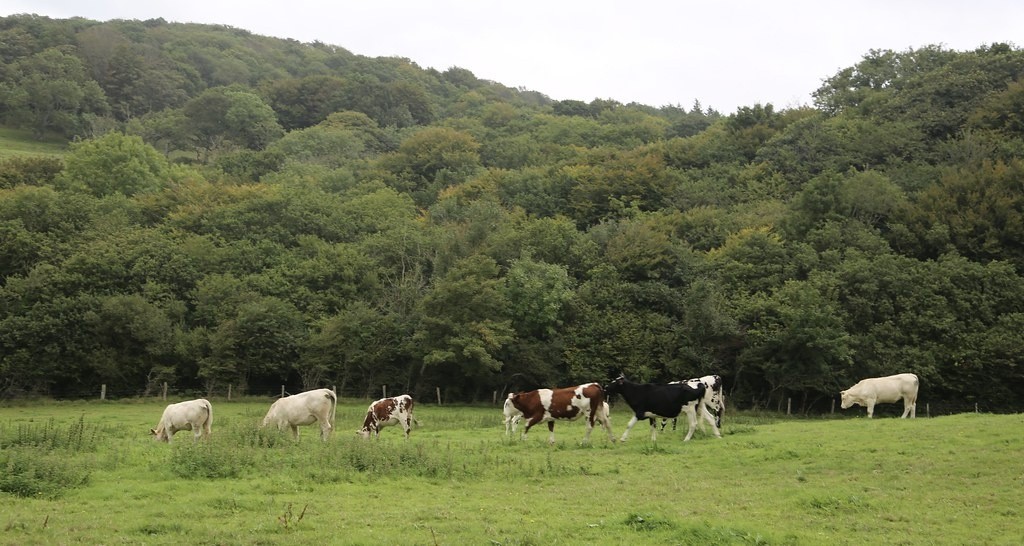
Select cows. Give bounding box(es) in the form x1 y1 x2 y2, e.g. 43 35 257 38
503 382 616 445
263 388 337 442
604 372 725 442
148 399 213 443
356 394 423 440
839 373 919 418
